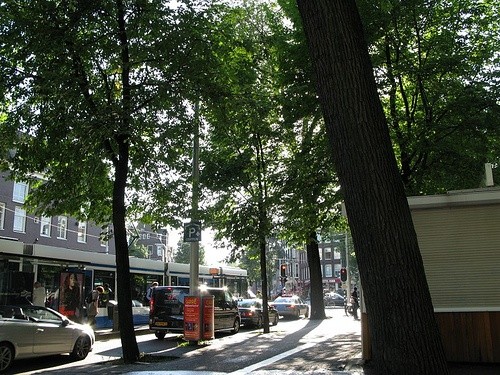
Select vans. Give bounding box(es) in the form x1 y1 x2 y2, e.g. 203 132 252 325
270 294 309 320
149 285 242 339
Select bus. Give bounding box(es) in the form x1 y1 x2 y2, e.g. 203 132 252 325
0 236 253 331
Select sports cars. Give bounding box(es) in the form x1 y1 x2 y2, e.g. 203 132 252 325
0 306 96 375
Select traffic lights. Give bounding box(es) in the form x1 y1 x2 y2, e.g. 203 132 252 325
340 267 347 282
281 264 286 282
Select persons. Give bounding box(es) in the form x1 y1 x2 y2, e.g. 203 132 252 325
145 282 158 304
61 273 82 322
86 286 104 334
352 287 361 321
32 282 46 307
256 291 262 299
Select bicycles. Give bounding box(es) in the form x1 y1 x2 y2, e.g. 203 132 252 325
345 296 361 317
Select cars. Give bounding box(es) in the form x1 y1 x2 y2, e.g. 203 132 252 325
236 298 279 329
323 293 347 307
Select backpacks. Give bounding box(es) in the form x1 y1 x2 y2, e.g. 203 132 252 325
87 291 97 304
